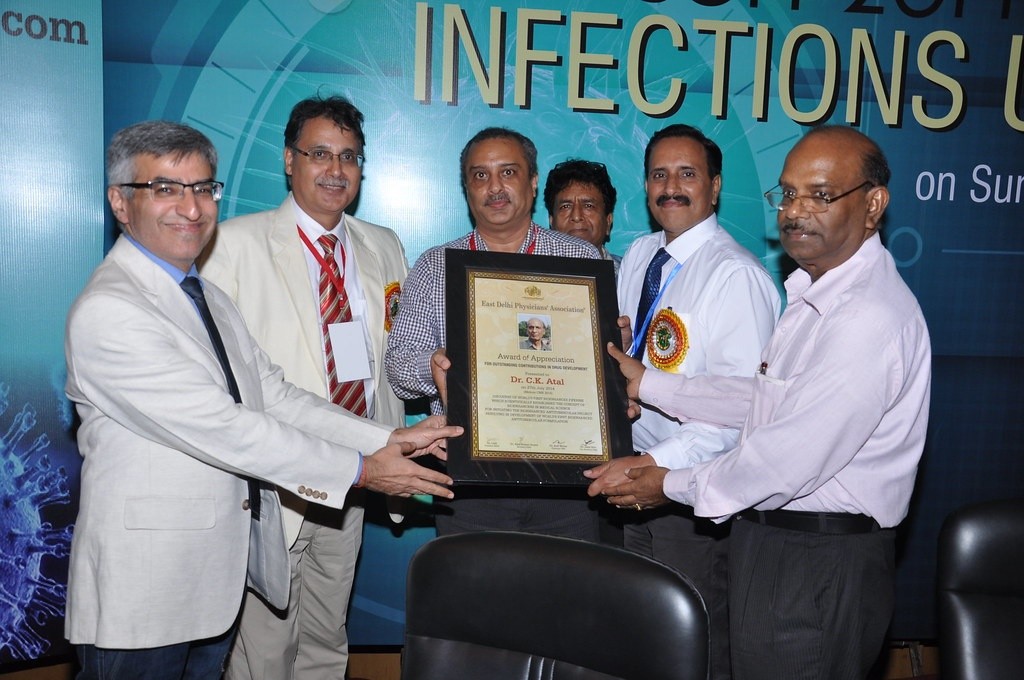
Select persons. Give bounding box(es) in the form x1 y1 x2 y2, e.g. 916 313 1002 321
583 124 782 680
195 84 409 680
384 127 603 540
601 124 928 680
63 122 465 680
544 158 623 291
519 318 552 351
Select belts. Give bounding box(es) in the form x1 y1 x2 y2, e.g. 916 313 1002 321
733 508 890 535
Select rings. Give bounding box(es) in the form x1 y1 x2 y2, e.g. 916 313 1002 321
634 503 641 511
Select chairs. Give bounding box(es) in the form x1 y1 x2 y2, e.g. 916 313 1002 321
935 498 1024 680
399 529 712 680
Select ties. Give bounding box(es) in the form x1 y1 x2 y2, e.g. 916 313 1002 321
629 247 671 367
178 276 263 521
316 233 368 421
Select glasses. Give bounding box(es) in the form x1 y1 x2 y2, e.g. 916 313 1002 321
118 181 223 202
290 145 365 167
762 180 876 213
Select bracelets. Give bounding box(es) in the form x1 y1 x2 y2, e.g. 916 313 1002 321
353 456 366 488
